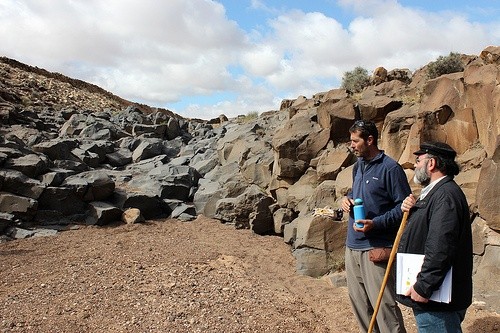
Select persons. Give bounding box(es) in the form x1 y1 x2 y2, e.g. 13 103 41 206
341 119 413 333
394 141 473 333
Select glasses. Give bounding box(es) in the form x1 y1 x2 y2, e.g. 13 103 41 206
355 121 371 135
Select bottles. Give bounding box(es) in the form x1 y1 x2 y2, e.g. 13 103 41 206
352 199 366 228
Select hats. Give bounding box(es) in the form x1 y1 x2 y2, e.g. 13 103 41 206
413 141 457 160
